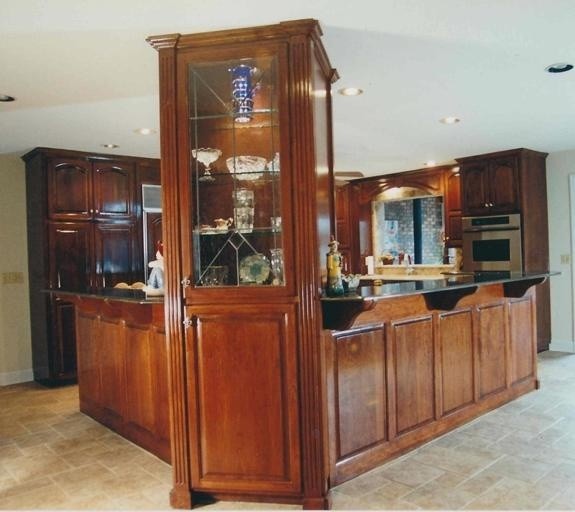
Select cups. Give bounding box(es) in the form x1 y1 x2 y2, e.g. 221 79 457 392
232 188 254 234
271 249 285 284
271 216 281 231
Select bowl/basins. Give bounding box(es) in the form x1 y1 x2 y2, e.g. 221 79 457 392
227 156 265 179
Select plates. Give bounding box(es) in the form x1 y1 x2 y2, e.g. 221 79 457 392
240 253 270 284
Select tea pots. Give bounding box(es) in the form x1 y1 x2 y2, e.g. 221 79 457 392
225 64 264 123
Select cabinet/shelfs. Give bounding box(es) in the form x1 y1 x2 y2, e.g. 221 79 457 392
457 153 550 217
145 17 339 511
19 146 165 387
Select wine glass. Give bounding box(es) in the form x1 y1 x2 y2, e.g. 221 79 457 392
193 149 223 181
202 265 228 287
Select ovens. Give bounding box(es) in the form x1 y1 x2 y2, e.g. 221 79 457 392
461 213 524 272
